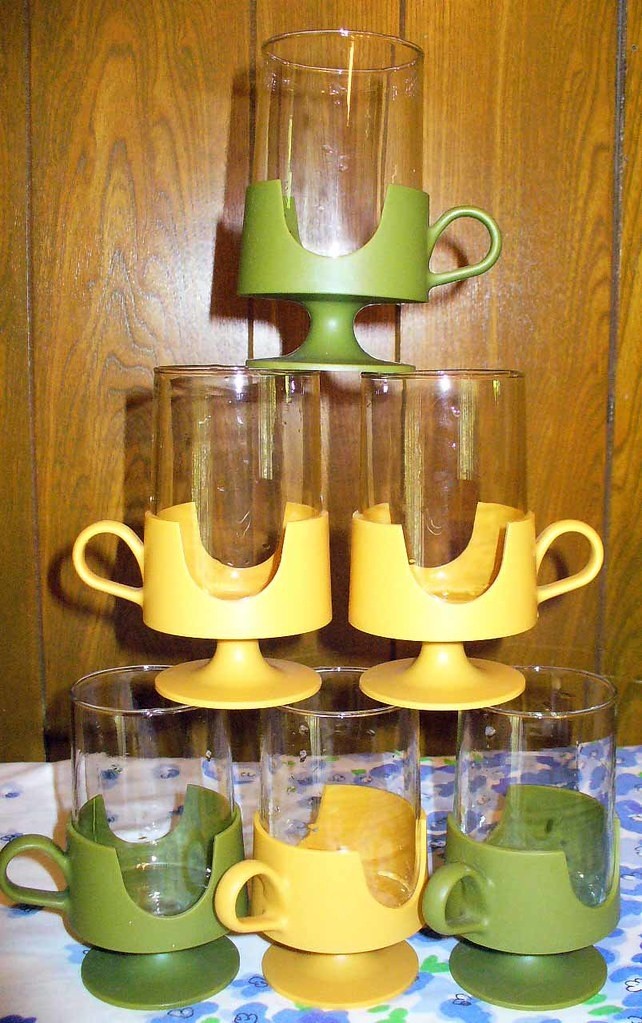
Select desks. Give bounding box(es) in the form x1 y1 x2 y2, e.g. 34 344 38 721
0 747 642 1023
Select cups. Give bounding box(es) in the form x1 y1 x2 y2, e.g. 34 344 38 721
0 664 248 1009
347 368 604 709
72 363 331 710
421 664 621 1011
215 663 430 1006
236 29 500 372
